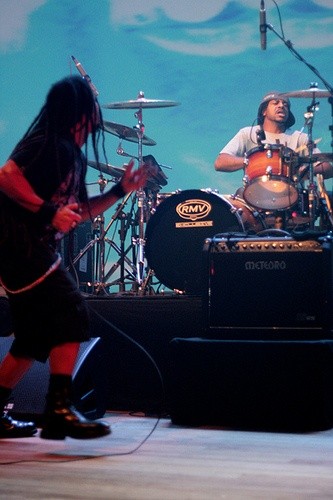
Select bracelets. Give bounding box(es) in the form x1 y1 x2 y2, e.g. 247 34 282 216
111 183 126 199
35 199 59 225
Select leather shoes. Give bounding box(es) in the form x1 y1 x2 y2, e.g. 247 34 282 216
39 402 112 441
0 413 38 438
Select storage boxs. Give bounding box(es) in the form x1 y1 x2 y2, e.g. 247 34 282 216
168 336 333 435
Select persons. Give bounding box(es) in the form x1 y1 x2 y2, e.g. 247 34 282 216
214 91 333 235
0 76 148 441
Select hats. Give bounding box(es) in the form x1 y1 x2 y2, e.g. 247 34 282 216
261 91 295 127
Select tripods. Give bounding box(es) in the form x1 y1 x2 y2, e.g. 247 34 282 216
64 104 156 295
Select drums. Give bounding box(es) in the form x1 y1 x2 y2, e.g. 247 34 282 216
143 188 267 295
135 190 176 221
241 144 304 211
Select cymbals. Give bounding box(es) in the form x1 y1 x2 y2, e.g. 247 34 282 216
103 120 158 146
276 88 332 98
86 159 125 178
100 98 179 109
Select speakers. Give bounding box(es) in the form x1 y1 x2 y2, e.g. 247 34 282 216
0 337 107 420
207 233 333 338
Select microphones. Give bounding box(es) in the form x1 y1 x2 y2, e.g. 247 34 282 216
258 117 266 140
70 56 99 96
259 0 267 50
